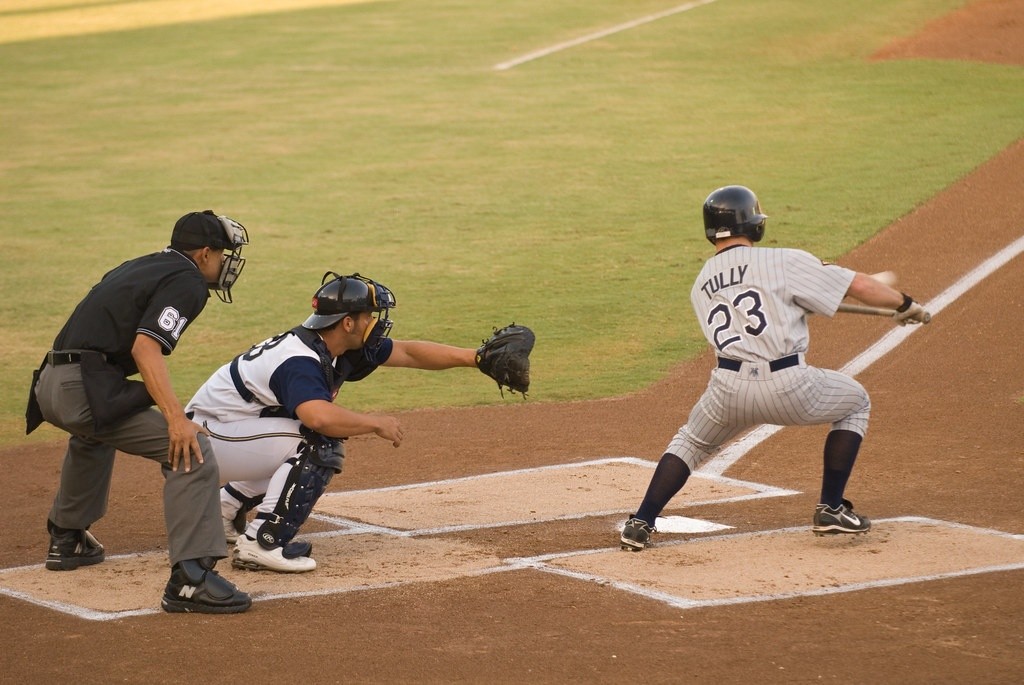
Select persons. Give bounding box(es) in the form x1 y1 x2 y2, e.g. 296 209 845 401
620 185 925 553
26 209 253 614
184 274 535 574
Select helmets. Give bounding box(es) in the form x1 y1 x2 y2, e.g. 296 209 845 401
703 185 769 246
302 276 379 330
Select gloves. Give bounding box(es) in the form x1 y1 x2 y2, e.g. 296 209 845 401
893 296 923 327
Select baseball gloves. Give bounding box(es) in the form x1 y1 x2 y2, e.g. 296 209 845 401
475 320 537 400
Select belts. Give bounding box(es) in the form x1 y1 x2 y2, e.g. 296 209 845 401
186 412 194 420
46 352 81 365
718 355 798 373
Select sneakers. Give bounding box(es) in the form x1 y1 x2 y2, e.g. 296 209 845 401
620 514 658 552
231 534 317 574
221 516 241 542
812 497 871 537
46 519 105 571
161 559 252 613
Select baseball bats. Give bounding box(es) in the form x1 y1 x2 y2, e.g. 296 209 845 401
837 302 931 325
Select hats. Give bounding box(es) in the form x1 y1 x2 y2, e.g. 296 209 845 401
170 209 241 251
